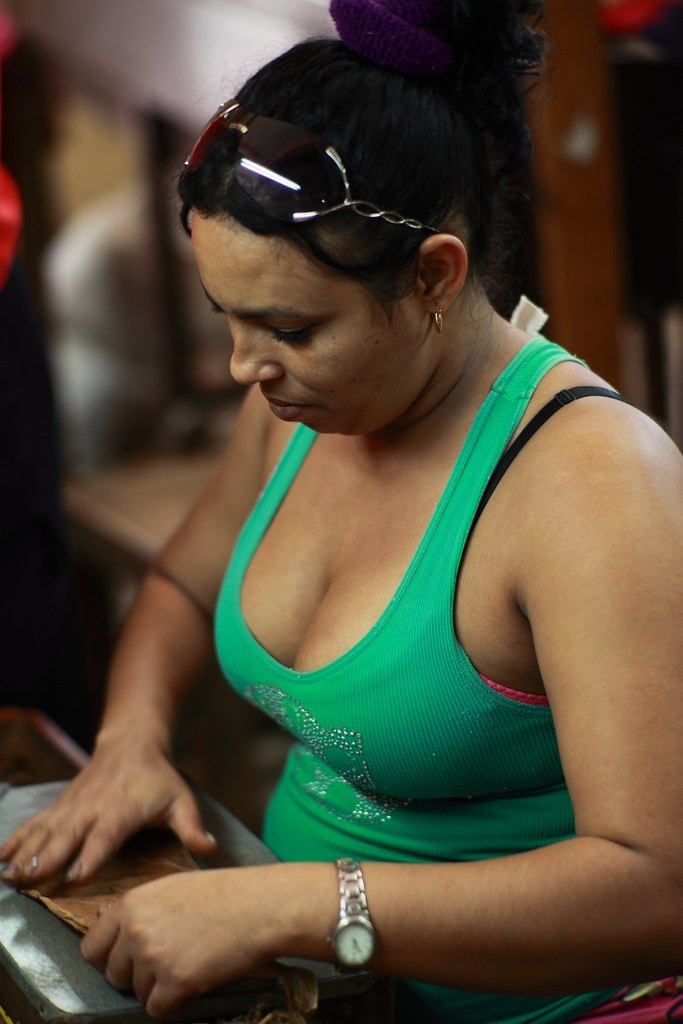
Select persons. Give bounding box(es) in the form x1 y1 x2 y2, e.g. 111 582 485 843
0 1 683 1023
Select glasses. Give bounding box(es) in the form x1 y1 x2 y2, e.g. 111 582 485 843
184 98 440 237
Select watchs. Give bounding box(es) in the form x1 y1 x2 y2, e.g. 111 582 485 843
327 856 379 975
